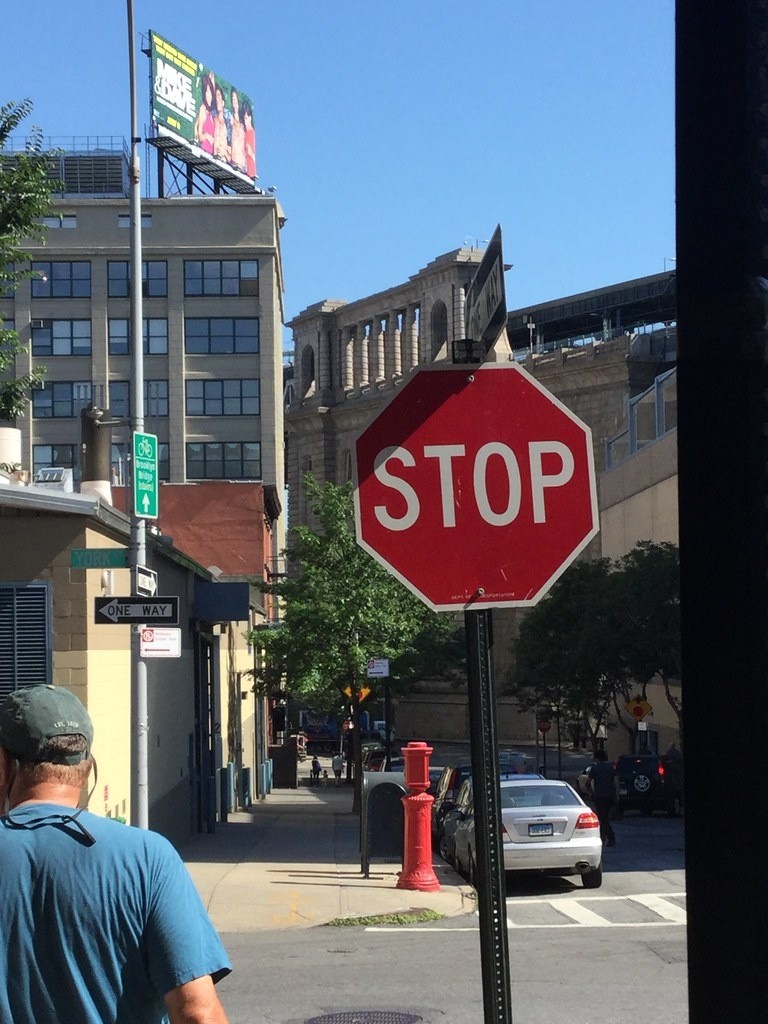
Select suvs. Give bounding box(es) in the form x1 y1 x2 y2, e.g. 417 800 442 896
615 753 683 814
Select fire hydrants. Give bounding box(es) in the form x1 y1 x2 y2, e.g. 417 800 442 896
395 740 442 892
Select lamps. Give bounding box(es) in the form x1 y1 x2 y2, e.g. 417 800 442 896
188 138 277 192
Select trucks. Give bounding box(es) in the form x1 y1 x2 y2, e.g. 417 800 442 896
288 728 308 762
301 711 396 755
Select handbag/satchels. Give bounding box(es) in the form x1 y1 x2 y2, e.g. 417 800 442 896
316 763 321 771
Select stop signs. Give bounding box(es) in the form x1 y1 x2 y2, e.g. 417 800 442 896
350 361 599 610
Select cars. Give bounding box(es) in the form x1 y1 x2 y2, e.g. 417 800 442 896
428 766 445 795
441 772 547 862
577 762 620 801
449 780 605 892
360 741 391 772
431 757 519 848
498 747 536 772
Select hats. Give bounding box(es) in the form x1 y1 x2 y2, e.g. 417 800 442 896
0 684 93 764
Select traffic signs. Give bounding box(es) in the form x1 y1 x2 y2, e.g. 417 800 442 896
70 547 132 569
94 597 179 626
133 565 158 597
131 429 159 518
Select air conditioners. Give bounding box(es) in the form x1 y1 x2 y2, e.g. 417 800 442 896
32 380 44 389
31 319 43 328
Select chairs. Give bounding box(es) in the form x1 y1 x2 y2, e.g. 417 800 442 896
541 794 564 806
502 797 515 808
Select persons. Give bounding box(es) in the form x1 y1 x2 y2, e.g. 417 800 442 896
322 770 328 787
639 742 653 755
332 752 343 787
0 684 232 1024
586 750 620 847
194 72 257 177
667 743 680 755
311 755 322 785
